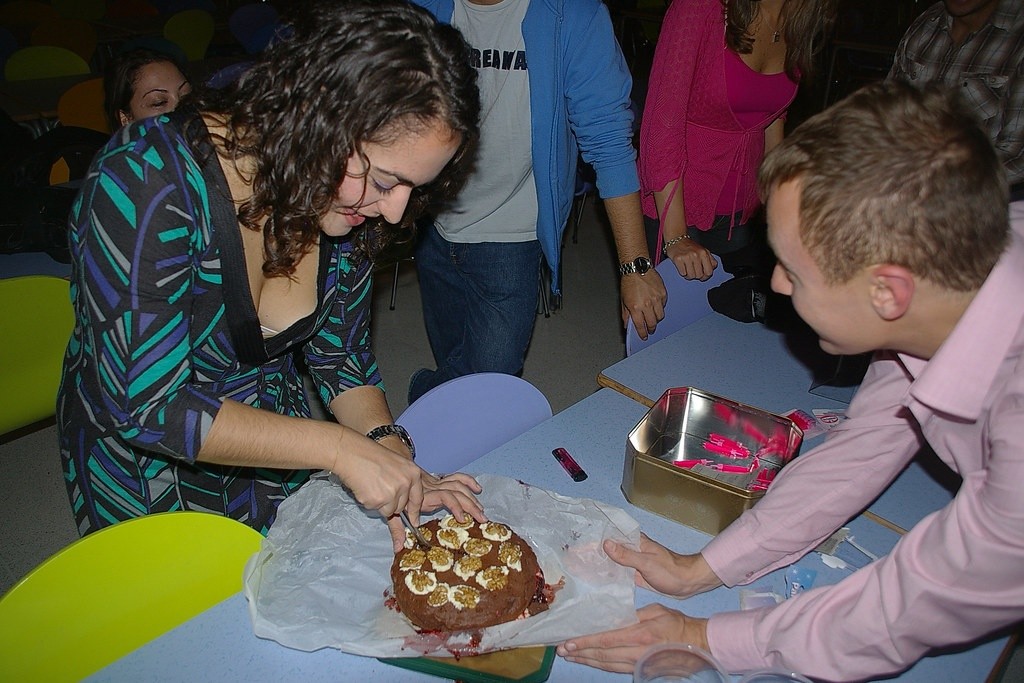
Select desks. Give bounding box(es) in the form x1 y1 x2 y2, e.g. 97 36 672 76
620 6 667 55
92 5 236 59
79 384 1023 683
0 51 260 134
595 306 965 528
822 24 910 113
0 16 128 49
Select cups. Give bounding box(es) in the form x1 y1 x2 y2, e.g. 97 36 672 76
737 667 814 683
633 642 730 683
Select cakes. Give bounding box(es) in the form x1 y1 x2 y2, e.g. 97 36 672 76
390 512 541 633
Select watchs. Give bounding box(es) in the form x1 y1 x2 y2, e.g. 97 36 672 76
367 424 416 461
619 256 653 278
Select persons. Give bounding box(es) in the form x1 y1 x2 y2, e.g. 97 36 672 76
882 0 1024 187
55 0 487 556
637 0 839 281
406 0 668 406
554 85 1024 683
103 37 194 139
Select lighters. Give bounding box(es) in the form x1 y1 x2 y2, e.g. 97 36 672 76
553 448 590 483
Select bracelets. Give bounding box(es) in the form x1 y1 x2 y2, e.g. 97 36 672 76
663 234 691 255
329 426 344 474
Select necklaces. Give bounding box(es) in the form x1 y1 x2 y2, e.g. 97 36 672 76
761 9 788 43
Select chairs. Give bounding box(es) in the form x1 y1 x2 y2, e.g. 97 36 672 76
834 0 902 36
0 509 274 683
602 0 674 73
392 371 555 483
626 249 736 355
0 0 288 253
0 277 77 444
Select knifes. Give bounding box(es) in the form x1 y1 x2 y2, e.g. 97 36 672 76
399 510 430 549
